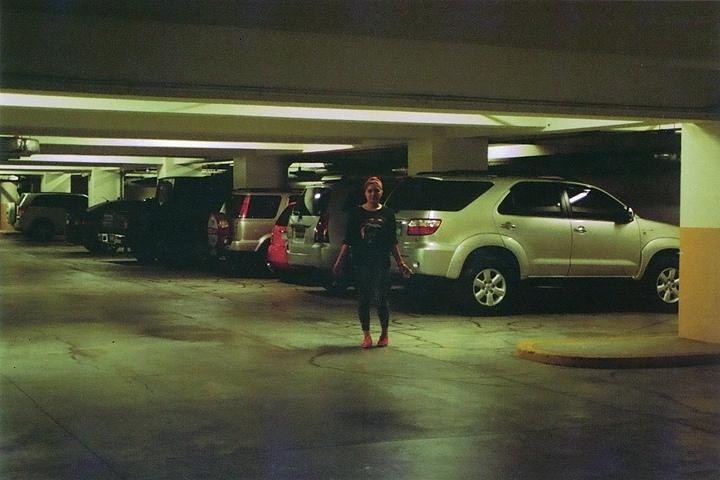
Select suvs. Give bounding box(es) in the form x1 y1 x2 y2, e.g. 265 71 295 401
372 168 680 317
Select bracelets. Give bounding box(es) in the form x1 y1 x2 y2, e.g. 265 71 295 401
398 262 405 267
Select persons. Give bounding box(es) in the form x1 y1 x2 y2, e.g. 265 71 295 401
332 173 416 349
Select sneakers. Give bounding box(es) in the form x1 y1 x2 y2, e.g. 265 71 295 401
362 337 372 348
377 336 387 347
